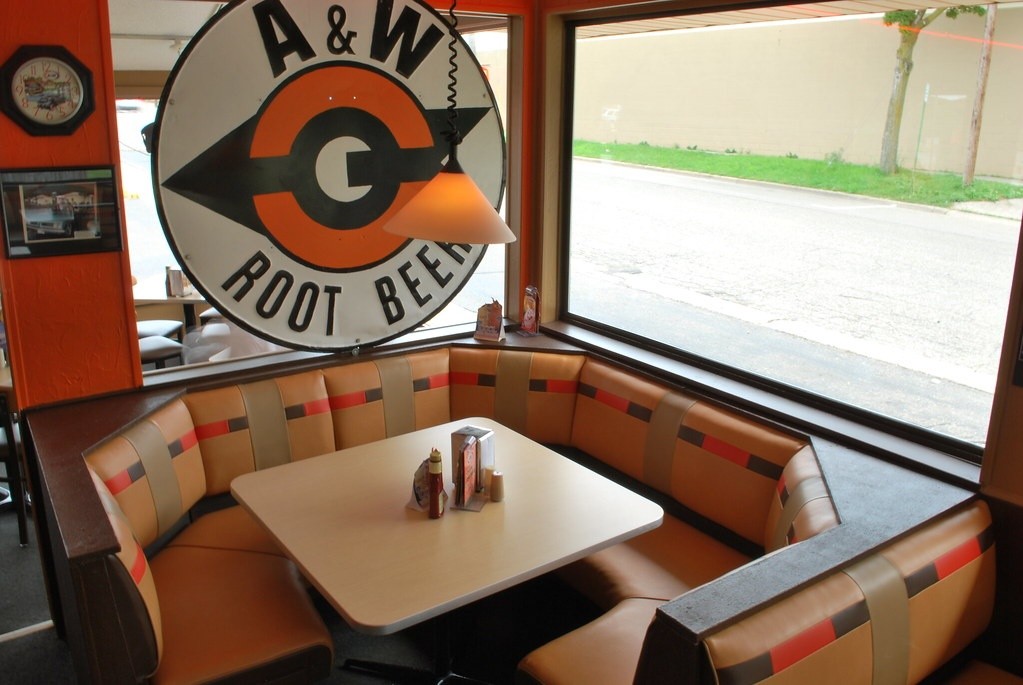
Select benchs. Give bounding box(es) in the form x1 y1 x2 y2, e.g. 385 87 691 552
85 347 839 685
700 498 1023 685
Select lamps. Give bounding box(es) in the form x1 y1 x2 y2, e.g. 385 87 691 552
383 0 517 245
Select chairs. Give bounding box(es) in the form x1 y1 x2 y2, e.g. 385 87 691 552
199 307 222 326
137 320 184 344
139 335 186 369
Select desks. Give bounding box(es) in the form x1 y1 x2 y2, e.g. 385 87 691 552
134 275 206 334
229 417 664 685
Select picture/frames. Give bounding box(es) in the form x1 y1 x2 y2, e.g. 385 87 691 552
0 164 125 259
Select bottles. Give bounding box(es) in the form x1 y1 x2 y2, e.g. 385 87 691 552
490 471 504 502
429 452 444 518
482 466 496 498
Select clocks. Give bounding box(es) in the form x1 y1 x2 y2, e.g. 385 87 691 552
0 45 96 137
